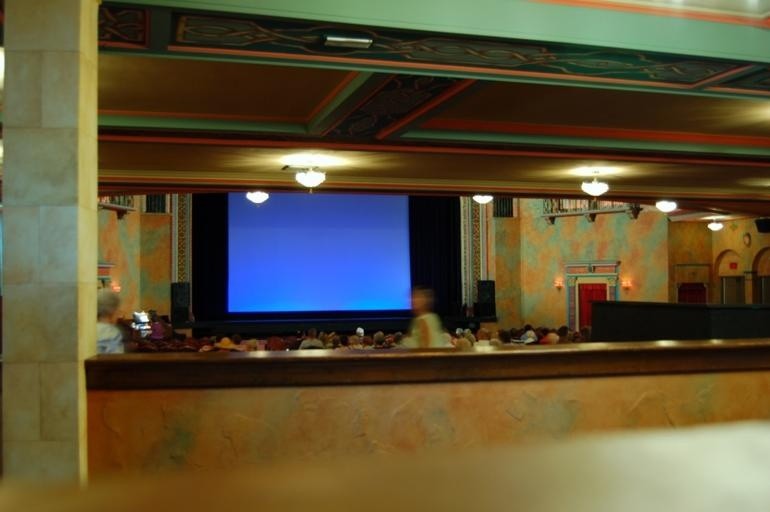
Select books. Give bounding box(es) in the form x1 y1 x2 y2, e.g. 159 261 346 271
98 194 135 207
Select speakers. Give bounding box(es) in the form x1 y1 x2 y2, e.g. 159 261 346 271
478 281 495 317
171 282 190 328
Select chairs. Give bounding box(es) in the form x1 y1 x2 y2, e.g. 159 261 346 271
114 314 411 358
489 319 588 348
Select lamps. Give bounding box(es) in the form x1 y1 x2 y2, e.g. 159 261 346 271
294 164 328 197
578 171 611 199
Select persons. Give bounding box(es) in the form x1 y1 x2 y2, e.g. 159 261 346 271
400 287 595 349
98 287 408 353
